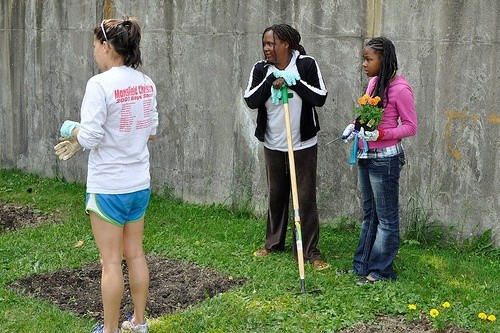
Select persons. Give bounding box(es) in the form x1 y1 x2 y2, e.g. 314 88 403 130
53 15 159 333
342 36 417 285
243 24 330 271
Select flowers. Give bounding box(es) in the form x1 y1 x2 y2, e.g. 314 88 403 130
354 95 384 128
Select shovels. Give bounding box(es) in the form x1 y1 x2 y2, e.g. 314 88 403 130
280 84 322 296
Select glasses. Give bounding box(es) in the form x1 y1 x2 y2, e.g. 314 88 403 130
100 18 109 44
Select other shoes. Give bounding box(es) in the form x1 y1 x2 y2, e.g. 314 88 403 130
357 277 379 286
338 267 357 278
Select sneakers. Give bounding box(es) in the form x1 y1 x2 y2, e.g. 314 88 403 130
123 315 149 333
93 325 122 333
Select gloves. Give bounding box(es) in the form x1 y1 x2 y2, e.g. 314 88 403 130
273 70 300 85
342 123 357 143
60 120 78 137
359 127 379 140
53 137 81 160
271 84 284 105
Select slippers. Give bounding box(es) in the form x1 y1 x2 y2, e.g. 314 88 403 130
254 247 270 256
313 259 330 271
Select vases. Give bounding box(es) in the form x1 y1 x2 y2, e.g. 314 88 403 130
355 115 371 131
